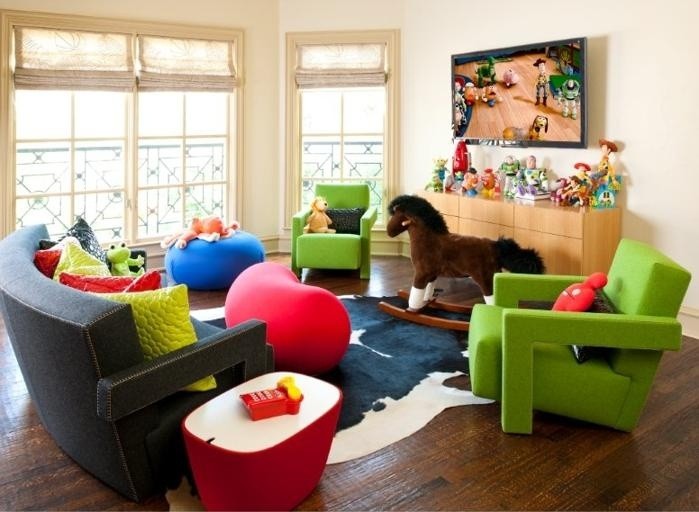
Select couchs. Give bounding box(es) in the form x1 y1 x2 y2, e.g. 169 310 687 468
0 217 280 508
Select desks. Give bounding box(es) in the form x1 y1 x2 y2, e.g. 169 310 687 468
181 364 344 512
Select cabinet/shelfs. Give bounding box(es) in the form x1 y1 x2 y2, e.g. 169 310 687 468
459 194 514 243
514 200 626 279
415 188 459 236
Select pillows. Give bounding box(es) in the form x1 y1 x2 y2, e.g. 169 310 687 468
54 240 111 287
568 287 621 366
57 212 106 264
59 267 162 296
84 282 220 394
38 239 58 251
323 206 367 234
31 236 80 278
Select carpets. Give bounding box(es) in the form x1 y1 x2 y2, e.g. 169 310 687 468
187 292 498 465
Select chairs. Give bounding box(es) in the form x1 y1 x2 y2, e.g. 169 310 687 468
288 182 379 282
461 237 694 443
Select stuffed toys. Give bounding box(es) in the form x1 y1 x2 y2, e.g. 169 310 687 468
549 272 609 313
105 242 146 279
303 196 337 235
424 138 623 210
161 214 241 248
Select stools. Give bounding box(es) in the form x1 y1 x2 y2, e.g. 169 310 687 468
164 233 266 289
223 262 352 373
